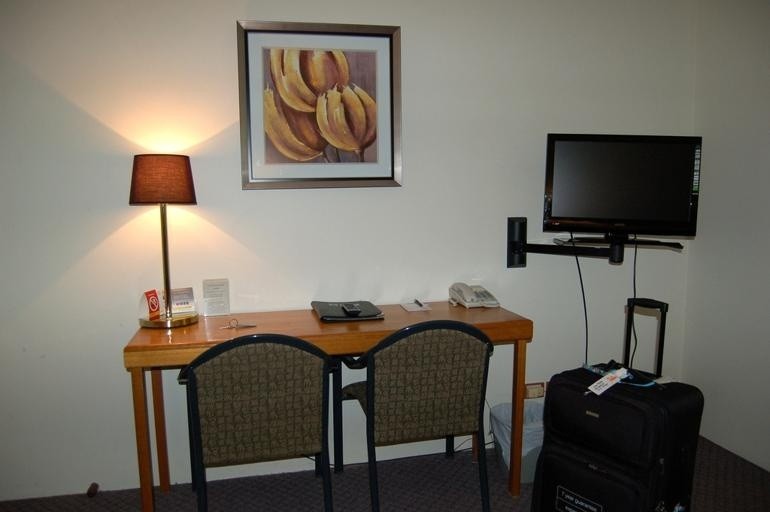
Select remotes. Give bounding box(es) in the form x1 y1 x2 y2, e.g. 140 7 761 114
342 302 361 314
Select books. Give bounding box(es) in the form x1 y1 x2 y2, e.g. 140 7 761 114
310 301 385 322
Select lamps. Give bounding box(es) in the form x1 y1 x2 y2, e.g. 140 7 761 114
129 154 199 327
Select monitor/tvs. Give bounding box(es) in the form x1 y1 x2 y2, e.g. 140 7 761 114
542 134 702 236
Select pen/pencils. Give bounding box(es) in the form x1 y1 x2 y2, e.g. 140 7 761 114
415 299 423 308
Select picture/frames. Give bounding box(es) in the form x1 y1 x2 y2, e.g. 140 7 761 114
236 19 402 190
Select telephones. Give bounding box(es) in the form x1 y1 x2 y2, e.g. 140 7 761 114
448 282 500 310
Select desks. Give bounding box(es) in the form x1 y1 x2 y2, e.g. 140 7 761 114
124 301 532 510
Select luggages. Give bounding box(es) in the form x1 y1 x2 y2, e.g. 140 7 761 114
531 295 704 512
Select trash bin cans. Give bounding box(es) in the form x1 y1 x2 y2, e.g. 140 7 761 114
489 399 543 484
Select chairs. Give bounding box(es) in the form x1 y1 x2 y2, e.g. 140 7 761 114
333 320 494 511
177 333 338 511
